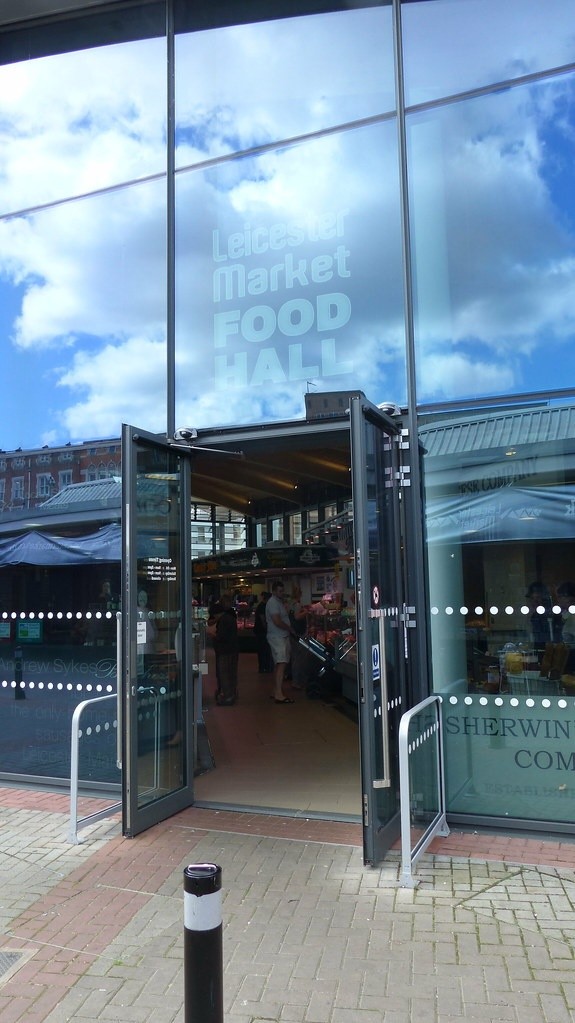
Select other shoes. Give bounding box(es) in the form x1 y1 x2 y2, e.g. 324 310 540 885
217 700 233 706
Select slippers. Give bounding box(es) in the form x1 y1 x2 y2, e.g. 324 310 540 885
270 695 276 699
274 697 293 704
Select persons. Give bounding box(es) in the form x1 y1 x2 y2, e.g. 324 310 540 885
252 591 272 674
265 582 297 705
524 581 575 675
207 595 238 706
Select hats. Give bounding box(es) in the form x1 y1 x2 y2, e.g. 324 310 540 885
525 581 549 598
556 581 575 596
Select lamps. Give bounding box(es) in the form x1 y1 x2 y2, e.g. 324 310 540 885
324 523 331 533
314 528 320 538
304 532 310 541
336 518 344 528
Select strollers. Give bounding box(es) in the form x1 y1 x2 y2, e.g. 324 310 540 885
295 635 341 700
